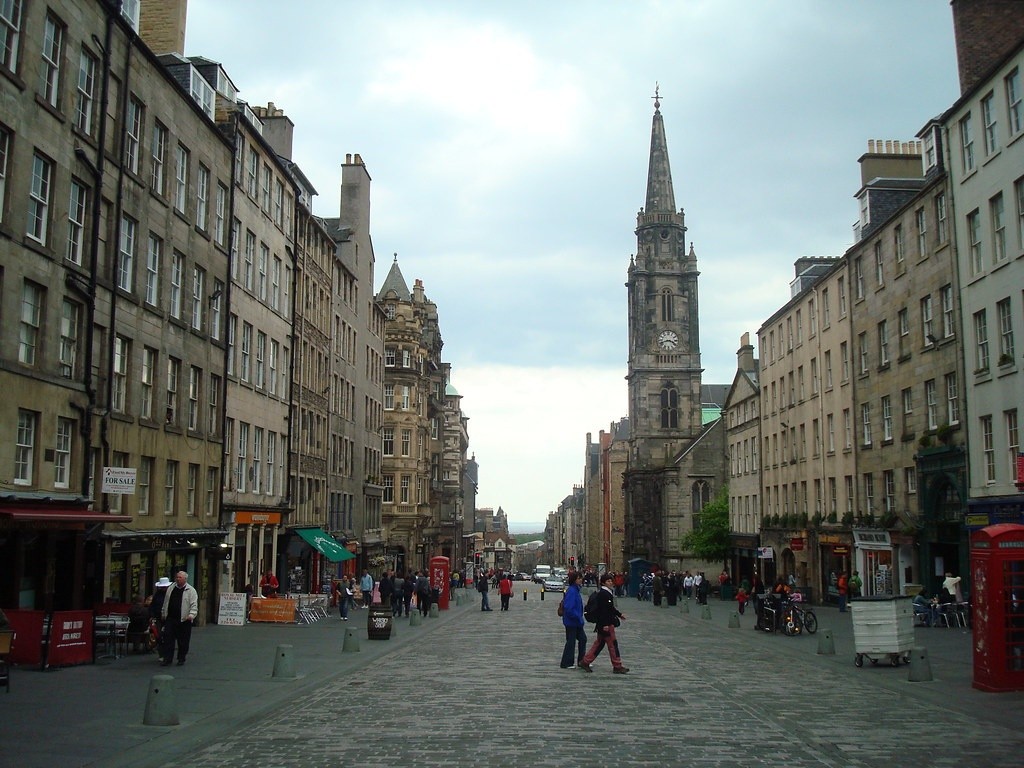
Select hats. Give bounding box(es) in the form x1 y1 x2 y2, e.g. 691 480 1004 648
155 577 173 587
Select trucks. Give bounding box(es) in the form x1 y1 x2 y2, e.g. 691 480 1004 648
553 567 564 576
531 565 552 584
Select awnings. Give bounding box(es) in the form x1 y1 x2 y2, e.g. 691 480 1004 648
0 508 134 537
296 528 356 561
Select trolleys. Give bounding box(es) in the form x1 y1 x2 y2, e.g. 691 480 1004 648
849 591 915 667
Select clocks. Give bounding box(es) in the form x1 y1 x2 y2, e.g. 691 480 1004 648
657 330 679 352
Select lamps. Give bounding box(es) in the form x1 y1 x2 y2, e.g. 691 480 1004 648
175 537 184 544
187 536 194 544
926 334 939 348
781 422 787 428
208 290 222 309
321 387 331 398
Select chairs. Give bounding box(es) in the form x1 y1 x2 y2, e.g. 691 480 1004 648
911 602 969 629
295 596 326 625
93 612 131 662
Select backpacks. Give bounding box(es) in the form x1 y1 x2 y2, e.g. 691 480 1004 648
850 577 858 590
584 590 602 624
834 578 842 590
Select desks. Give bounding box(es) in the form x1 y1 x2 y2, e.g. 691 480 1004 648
98 622 130 660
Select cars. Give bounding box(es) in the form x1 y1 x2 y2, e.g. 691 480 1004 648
542 577 566 592
555 570 569 586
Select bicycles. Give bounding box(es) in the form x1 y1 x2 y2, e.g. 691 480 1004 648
781 594 818 636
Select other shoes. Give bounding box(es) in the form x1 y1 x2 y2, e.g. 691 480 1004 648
487 608 493 611
613 666 629 674
177 660 184 665
340 617 343 620
578 663 593 667
344 617 348 620
840 608 848 612
160 659 172 665
482 608 487 611
930 623 936 627
579 660 593 672
361 605 369 609
566 665 575 668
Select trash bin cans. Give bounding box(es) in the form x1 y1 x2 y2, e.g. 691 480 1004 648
851 594 914 668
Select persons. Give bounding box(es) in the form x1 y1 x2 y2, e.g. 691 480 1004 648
378 570 432 618
498 575 513 610
474 568 514 589
561 572 592 668
567 566 599 589
129 577 173 660
914 574 962 625
718 570 796 614
640 570 708 606
246 584 253 608
331 575 359 620
161 571 198 665
478 572 493 611
838 571 862 612
449 571 466 598
359 568 375 609
259 568 278 597
579 575 629 673
604 570 629 597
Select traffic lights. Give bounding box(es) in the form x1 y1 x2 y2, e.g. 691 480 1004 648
568 558 570 564
571 556 574 565
475 554 479 564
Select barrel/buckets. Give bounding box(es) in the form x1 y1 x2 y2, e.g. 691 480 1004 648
367 605 393 640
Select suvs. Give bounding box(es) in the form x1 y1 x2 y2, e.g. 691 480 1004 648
515 573 531 581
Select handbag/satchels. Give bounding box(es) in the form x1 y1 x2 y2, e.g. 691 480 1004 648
558 588 568 617
509 591 514 597
